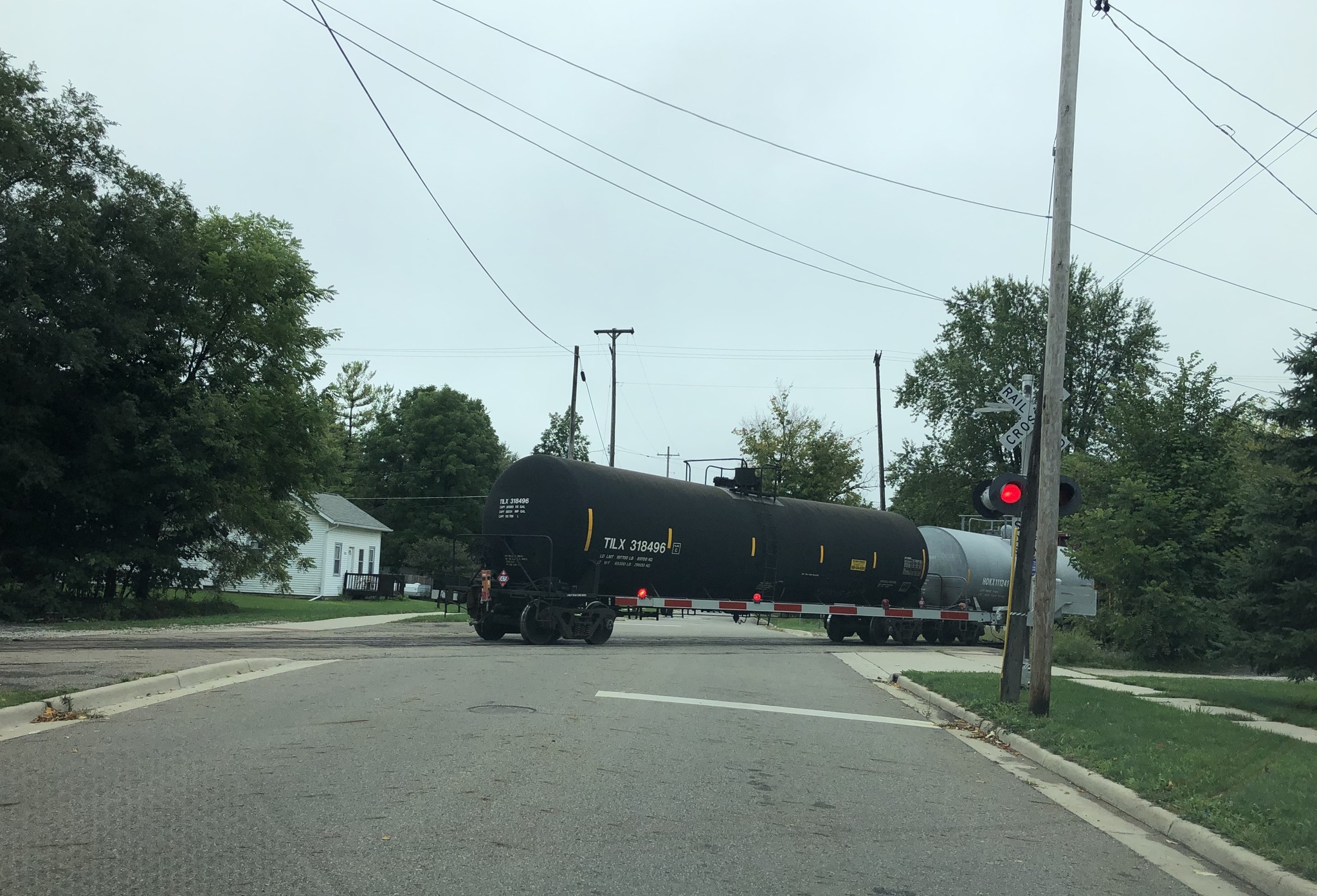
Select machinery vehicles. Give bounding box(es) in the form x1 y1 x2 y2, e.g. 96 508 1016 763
442 455 1099 647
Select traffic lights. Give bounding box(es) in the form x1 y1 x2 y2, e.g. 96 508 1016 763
1060 475 1083 518
991 473 1028 515
